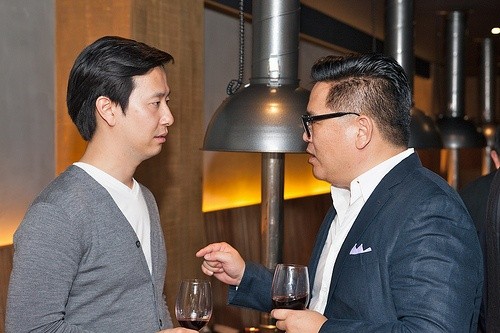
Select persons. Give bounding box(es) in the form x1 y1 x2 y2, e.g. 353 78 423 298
478 166 500 333
3 33 199 333
194 50 489 333
459 125 500 231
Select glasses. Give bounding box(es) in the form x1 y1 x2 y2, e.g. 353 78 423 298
301 109 360 138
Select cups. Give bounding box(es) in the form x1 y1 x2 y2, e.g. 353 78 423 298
270 263 310 312
174 278 212 333
